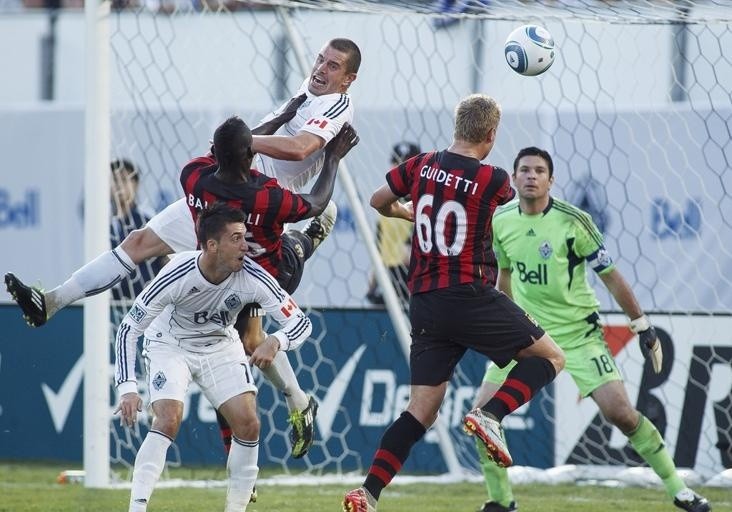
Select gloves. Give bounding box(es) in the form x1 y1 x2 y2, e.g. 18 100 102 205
628 313 664 376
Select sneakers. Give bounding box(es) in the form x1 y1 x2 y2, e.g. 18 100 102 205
340 488 370 511
300 197 338 256
477 497 519 511
3 271 49 329
460 406 515 468
671 485 711 512
248 485 258 504
286 392 319 459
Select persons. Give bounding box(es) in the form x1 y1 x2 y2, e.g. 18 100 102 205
179 92 359 503
4 38 361 459
341 96 567 512
113 201 313 512
109 159 171 302
365 139 422 307
470 145 711 512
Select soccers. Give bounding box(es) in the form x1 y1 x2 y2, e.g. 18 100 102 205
505 23 556 76
